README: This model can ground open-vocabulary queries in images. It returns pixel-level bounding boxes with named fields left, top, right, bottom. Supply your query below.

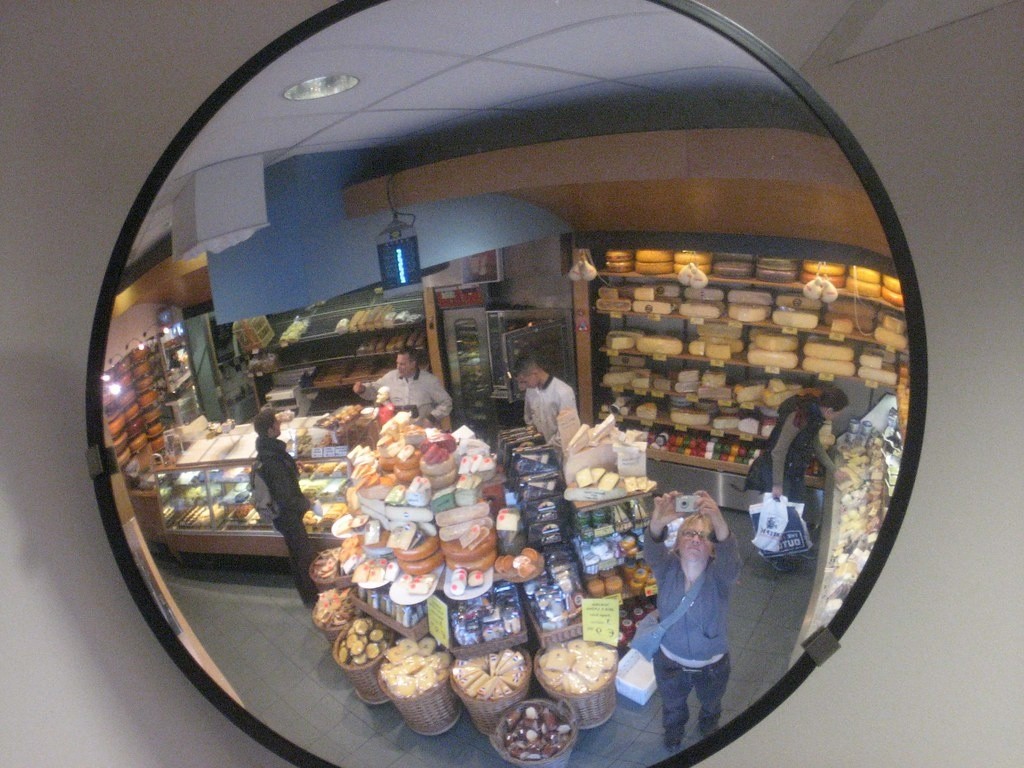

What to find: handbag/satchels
left=748, top=491, right=812, bottom=560
left=632, top=612, right=665, bottom=662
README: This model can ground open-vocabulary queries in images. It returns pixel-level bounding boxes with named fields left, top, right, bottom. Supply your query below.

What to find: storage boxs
left=617, top=448, right=647, bottom=477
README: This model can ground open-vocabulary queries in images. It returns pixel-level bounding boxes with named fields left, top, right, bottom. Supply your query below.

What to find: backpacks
left=249, top=452, right=285, bottom=523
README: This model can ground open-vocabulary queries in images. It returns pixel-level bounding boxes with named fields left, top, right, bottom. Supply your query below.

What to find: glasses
left=681, top=530, right=710, bottom=540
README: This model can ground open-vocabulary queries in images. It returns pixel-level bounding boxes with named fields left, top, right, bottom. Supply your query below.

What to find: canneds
left=844, top=418, right=873, bottom=446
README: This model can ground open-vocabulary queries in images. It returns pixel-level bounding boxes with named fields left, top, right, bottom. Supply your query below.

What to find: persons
left=232, top=319, right=273, bottom=403
left=169, top=351, right=181, bottom=370
left=254, top=410, right=319, bottom=606
left=353, top=347, right=453, bottom=426
left=744, top=387, right=849, bottom=573
left=644, top=489, right=738, bottom=751
left=514, top=356, right=581, bottom=448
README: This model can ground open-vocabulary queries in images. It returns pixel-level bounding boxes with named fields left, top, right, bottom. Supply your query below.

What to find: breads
left=278, top=304, right=426, bottom=356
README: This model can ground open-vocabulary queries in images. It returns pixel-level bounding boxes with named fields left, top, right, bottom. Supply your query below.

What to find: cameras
left=674, top=495, right=701, bottom=513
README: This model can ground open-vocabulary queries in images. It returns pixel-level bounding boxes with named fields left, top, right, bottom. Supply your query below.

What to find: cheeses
left=595, top=252, right=908, bottom=448
left=159, top=404, right=659, bottom=758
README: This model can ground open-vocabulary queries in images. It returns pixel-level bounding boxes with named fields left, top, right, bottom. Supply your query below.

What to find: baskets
left=308, top=552, right=619, bottom=768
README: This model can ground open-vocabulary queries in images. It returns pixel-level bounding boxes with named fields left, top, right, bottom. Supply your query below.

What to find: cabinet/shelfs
left=567, top=476, right=657, bottom=600
left=250, top=290, right=452, bottom=432
left=182, top=297, right=257, bottom=425
left=599, top=234, right=910, bottom=527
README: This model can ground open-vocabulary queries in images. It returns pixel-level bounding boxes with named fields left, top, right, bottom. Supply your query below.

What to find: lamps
left=377, top=175, right=425, bottom=300
